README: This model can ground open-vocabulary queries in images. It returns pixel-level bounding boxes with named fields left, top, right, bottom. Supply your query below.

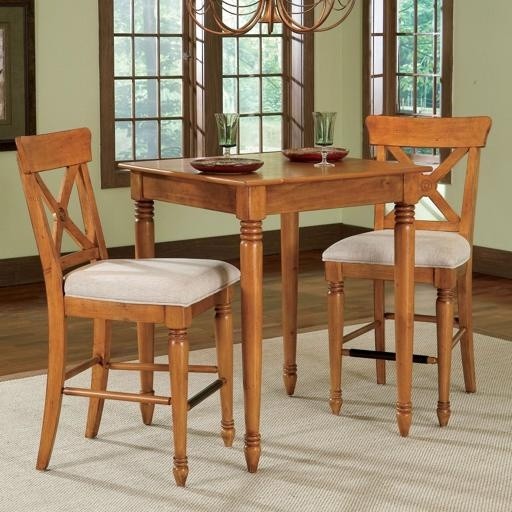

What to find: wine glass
left=312, top=111, right=336, bottom=169
left=215, top=111, right=240, bottom=156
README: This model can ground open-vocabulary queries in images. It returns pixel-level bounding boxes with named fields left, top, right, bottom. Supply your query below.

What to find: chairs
left=320, top=114, right=491, bottom=427
left=13, top=126, right=244, bottom=487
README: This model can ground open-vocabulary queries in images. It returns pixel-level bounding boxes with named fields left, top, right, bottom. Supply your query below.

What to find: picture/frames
left=0, top=1, right=35, bottom=151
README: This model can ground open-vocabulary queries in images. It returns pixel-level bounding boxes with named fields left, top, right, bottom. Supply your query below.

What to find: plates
left=190, top=157, right=264, bottom=175
left=281, top=146, right=350, bottom=162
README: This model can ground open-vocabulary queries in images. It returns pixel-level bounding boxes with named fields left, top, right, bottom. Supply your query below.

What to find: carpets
left=2, top=320, right=510, bottom=512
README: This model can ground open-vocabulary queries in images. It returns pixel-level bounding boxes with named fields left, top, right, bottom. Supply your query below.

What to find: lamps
left=180, top=0, right=358, bottom=39
